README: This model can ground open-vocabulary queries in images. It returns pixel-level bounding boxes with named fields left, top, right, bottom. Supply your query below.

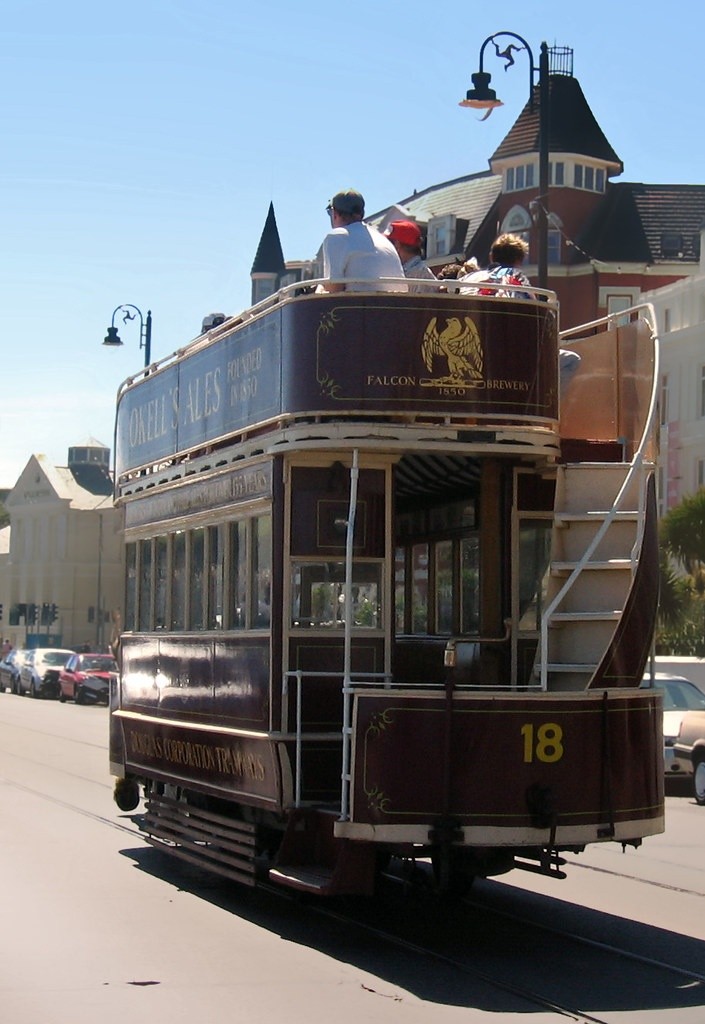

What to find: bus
left=110, top=277, right=663, bottom=899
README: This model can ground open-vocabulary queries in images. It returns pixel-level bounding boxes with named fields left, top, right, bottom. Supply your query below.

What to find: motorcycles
left=75, top=674, right=110, bottom=705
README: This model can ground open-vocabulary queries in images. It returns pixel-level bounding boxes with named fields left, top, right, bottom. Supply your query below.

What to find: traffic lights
left=0, top=603, right=59, bottom=626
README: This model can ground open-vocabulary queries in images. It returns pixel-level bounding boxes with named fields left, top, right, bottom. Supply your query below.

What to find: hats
left=382, top=220, right=420, bottom=248
left=325, top=189, right=365, bottom=217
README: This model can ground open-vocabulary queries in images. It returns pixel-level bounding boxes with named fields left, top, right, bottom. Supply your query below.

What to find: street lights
left=102, top=304, right=152, bottom=377
left=459, top=30, right=549, bottom=302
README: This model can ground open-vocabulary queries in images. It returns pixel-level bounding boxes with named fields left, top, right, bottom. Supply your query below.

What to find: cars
left=673, top=712, right=705, bottom=806
left=642, top=672, right=705, bottom=778
left=58, top=653, right=119, bottom=703
left=0, top=649, right=23, bottom=694
left=14, top=648, right=76, bottom=699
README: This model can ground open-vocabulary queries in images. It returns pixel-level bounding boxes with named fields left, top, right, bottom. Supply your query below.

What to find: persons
left=453, top=232, right=583, bottom=412
left=315, top=190, right=408, bottom=294
left=382, top=219, right=441, bottom=293
